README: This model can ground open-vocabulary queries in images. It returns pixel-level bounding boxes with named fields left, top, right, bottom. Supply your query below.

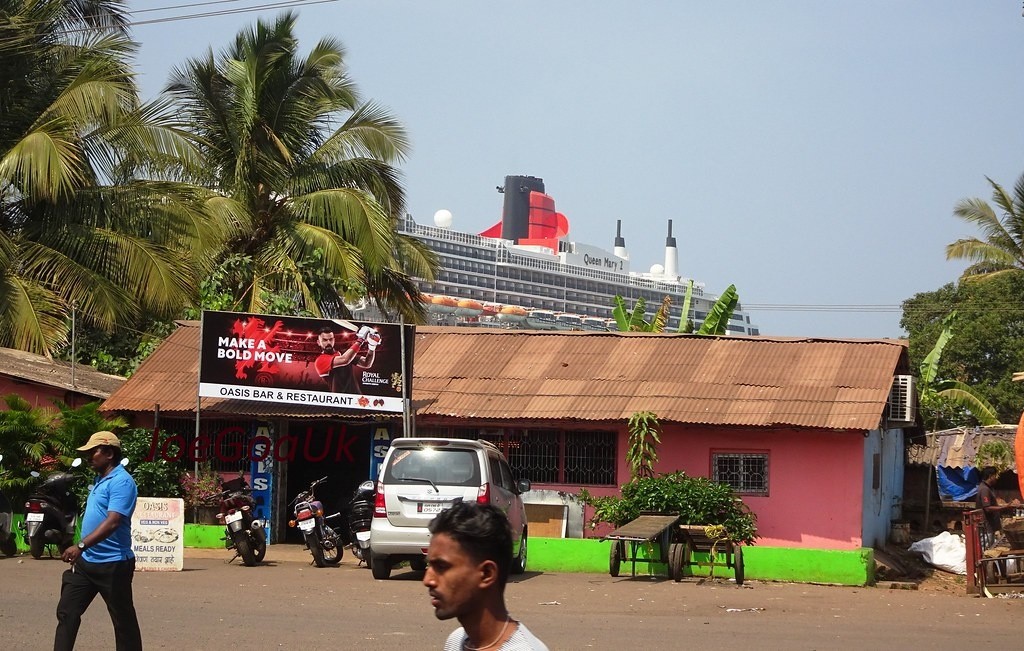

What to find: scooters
left=0, top=454, right=17, bottom=557
left=343, top=478, right=379, bottom=569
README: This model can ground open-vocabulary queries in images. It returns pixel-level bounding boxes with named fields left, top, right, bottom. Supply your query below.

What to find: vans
left=369, top=437, right=533, bottom=580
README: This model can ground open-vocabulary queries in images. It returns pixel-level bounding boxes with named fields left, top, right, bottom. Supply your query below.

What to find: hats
left=75, top=431, right=119, bottom=451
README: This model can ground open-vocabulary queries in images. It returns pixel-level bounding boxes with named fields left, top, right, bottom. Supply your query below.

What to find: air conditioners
left=884, top=375, right=916, bottom=423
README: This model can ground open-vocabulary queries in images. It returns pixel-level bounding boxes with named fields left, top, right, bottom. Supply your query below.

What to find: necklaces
left=463, top=613, right=511, bottom=651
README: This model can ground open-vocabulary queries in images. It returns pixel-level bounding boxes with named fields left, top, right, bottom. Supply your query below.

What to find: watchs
left=78, top=540, right=88, bottom=552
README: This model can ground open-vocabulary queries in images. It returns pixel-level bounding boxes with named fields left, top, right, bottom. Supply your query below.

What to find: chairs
left=402, top=465, right=472, bottom=484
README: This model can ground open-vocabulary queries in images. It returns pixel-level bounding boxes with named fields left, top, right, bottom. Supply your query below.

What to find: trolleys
left=599, top=510, right=681, bottom=579
left=673, top=523, right=746, bottom=586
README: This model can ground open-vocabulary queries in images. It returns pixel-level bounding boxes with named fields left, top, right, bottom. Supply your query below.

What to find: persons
left=976, top=466, right=1021, bottom=557
left=424, top=498, right=550, bottom=651
left=315, top=325, right=384, bottom=396
left=52, top=430, right=143, bottom=650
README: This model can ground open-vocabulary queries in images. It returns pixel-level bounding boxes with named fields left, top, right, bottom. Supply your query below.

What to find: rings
left=69, top=558, right=72, bottom=560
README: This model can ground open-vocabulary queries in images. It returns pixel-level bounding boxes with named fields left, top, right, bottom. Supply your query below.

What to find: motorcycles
left=17, top=457, right=82, bottom=560
left=288, top=475, right=344, bottom=569
left=205, top=454, right=268, bottom=566
